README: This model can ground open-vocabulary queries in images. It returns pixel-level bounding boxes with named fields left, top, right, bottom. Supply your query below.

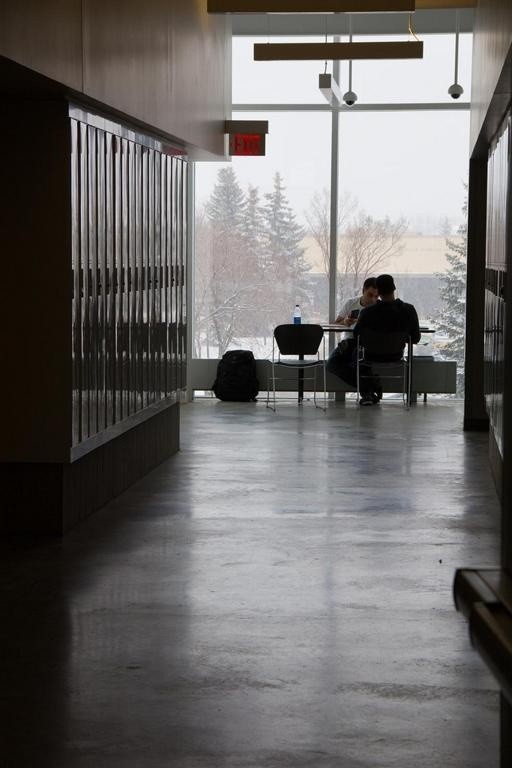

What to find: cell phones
left=351, top=310, right=359, bottom=324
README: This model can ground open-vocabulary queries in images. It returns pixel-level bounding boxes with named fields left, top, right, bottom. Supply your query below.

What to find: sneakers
left=359, top=391, right=380, bottom=406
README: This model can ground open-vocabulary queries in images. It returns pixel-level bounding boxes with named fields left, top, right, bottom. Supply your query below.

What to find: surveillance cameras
left=343, top=92, right=357, bottom=106
left=448, top=84, right=463, bottom=99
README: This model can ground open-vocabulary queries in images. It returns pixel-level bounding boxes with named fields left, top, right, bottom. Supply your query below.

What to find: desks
left=293, top=320, right=436, bottom=407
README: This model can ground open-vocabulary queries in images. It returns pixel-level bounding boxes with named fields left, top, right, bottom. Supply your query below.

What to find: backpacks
left=212, top=348, right=261, bottom=404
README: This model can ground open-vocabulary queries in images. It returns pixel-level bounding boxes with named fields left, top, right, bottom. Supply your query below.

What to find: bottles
left=293, top=305, right=302, bottom=325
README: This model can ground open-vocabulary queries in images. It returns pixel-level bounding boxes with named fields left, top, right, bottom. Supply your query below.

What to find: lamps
left=317, top=13, right=344, bottom=112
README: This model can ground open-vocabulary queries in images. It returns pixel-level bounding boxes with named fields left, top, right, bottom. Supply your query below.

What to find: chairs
left=265, top=323, right=328, bottom=414
left=353, top=320, right=413, bottom=409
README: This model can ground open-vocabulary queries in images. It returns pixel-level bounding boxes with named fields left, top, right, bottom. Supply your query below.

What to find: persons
left=328, top=275, right=421, bottom=405
left=334, top=278, right=378, bottom=344
